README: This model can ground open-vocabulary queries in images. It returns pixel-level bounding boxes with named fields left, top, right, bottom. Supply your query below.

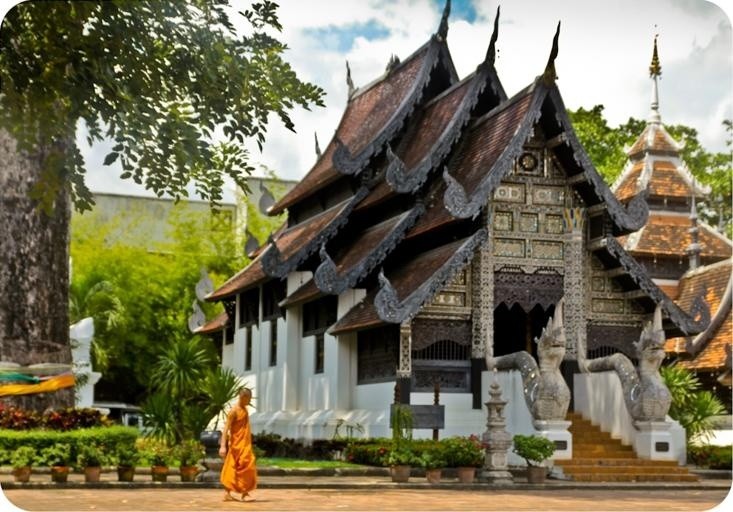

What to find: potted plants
left=10, top=439, right=206, bottom=482
left=388, top=433, right=558, bottom=484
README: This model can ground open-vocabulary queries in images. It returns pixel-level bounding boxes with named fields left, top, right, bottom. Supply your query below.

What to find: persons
left=219, top=388, right=257, bottom=502
